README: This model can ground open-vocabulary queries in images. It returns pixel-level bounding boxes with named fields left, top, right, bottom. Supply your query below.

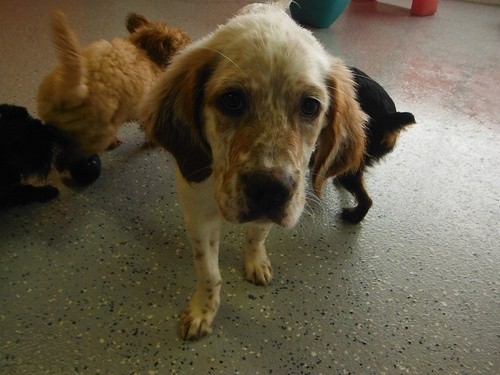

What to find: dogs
left=136, top=2, right=370, bottom=341
left=309, top=61, right=418, bottom=226
left=0, top=104, right=75, bottom=204
left=36, top=10, right=194, bottom=189
